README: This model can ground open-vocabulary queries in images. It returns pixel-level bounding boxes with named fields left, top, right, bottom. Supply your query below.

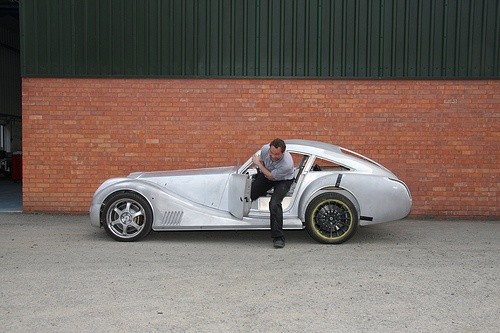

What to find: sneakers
left=274, top=238, right=285, bottom=248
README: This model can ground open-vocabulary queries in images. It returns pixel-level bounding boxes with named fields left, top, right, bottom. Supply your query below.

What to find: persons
left=251, top=138, right=296, bottom=249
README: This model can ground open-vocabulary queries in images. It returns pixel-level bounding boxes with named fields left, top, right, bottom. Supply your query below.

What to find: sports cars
left=89, top=138, right=413, bottom=245
